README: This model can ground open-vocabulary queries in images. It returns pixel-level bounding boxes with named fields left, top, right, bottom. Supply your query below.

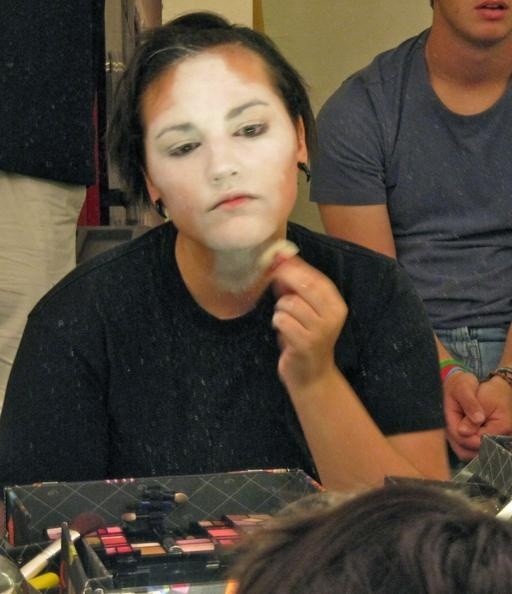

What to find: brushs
left=19, top=511, right=111, bottom=580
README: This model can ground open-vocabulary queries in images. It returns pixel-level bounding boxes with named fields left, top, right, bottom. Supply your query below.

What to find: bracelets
left=439, top=359, right=475, bottom=388
left=478, top=366, right=512, bottom=386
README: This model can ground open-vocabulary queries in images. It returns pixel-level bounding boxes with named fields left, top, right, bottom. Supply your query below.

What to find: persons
left=0, top=0, right=97, bottom=416
left=226, top=485, right=512, bottom=594
left=0, top=10, right=451, bottom=494
left=309, top=0, right=512, bottom=459
